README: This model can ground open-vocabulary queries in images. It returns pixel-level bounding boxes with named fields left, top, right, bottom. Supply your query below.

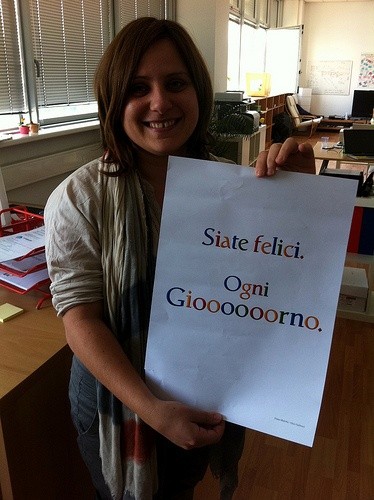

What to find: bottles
left=338, top=128, right=344, bottom=149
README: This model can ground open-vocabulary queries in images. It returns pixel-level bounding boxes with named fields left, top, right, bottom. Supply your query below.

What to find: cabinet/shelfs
left=0, top=205, right=53, bottom=310
left=248, top=92, right=294, bottom=150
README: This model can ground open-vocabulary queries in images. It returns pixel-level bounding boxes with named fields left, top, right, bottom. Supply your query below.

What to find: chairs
left=285, top=94, right=323, bottom=138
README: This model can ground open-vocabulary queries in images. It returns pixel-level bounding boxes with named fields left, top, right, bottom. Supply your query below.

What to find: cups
left=321, top=137, right=329, bottom=151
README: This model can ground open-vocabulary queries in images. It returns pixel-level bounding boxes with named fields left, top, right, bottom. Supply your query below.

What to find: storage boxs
left=339, top=267, right=369, bottom=312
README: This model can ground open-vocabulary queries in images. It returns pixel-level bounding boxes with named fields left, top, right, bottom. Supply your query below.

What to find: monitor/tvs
left=351, top=90, right=374, bottom=117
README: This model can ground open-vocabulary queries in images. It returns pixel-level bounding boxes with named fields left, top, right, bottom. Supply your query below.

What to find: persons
left=43, top=17, right=316, bottom=500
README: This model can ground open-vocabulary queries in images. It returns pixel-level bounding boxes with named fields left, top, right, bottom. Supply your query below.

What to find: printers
left=214, top=91, right=260, bottom=135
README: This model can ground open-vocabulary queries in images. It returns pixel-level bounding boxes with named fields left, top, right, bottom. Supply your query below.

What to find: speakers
left=343, top=125, right=374, bottom=154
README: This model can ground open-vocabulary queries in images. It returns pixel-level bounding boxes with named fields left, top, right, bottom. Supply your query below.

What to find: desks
left=317, top=117, right=369, bottom=129
left=213, top=125, right=266, bottom=168
left=313, top=142, right=374, bottom=175
left=0, top=280, right=101, bottom=500
